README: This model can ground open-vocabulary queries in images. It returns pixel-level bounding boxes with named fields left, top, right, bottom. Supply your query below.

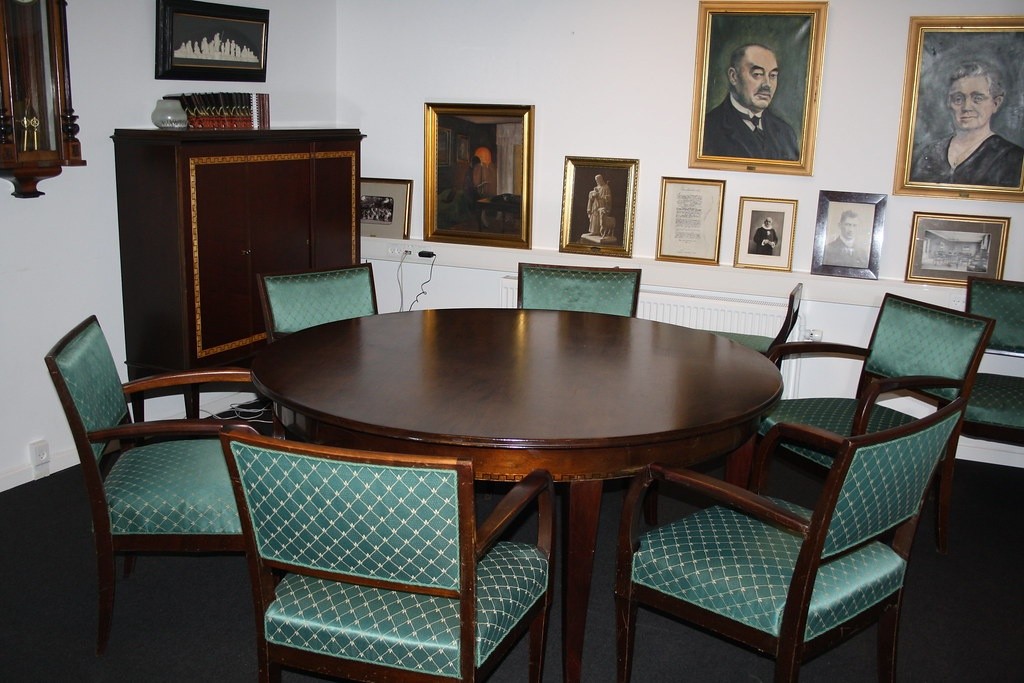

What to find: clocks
left=0, top=0, right=91, bottom=198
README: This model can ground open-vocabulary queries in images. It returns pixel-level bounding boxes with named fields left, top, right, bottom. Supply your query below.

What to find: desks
left=249, top=308, right=783, bottom=683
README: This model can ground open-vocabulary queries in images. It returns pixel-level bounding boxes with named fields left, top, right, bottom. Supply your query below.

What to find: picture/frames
left=689, top=0, right=829, bottom=176
left=810, top=190, right=887, bottom=279
left=906, top=211, right=1010, bottom=289
left=733, top=196, right=798, bottom=272
left=891, top=16, right=1024, bottom=204
left=422, top=103, right=536, bottom=251
left=360, top=177, right=414, bottom=239
left=559, top=156, right=640, bottom=258
left=155, top=0, right=269, bottom=82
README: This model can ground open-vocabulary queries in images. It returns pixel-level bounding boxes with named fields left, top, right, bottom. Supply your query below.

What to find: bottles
left=151, top=99, right=187, bottom=129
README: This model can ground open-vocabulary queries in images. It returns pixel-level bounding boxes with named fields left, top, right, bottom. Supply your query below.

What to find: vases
left=152, top=100, right=188, bottom=128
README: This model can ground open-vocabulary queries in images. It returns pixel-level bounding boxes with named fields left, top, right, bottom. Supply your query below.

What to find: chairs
left=46, top=262, right=1024, bottom=683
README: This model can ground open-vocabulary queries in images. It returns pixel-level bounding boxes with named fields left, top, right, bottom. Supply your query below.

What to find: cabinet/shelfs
left=110, top=128, right=366, bottom=398
